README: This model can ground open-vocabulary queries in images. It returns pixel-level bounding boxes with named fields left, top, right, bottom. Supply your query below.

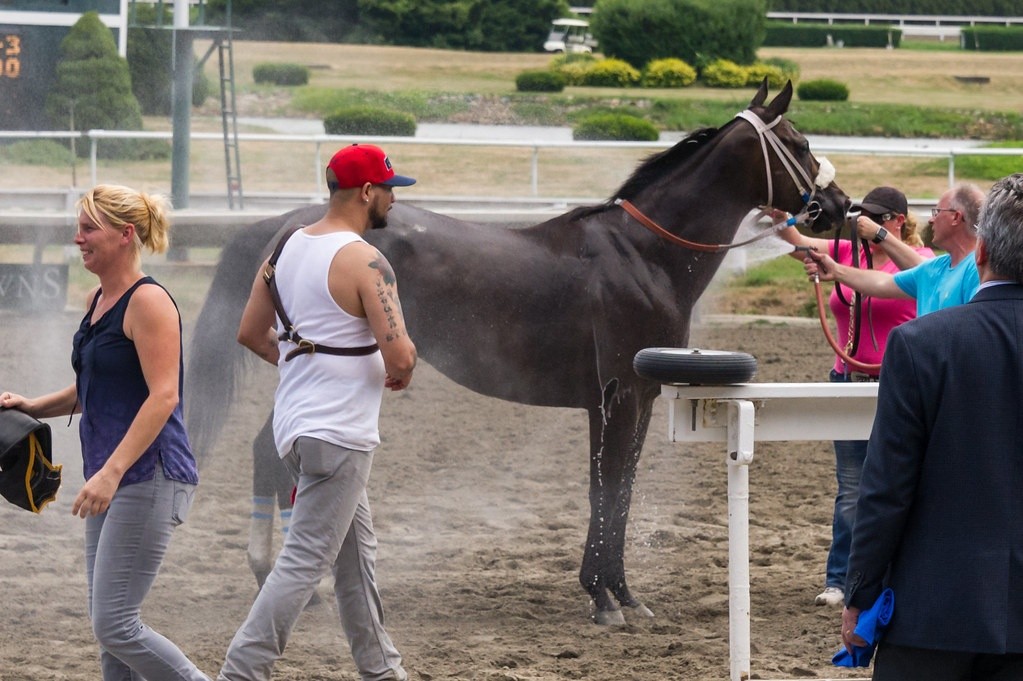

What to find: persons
left=0, top=183, right=213, bottom=681
left=842, top=172, right=1023, bottom=681
left=215, top=144, right=417, bottom=681
left=765, top=184, right=981, bottom=602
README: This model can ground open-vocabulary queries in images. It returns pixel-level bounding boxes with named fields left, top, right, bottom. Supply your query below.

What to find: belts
left=830, top=371, right=880, bottom=382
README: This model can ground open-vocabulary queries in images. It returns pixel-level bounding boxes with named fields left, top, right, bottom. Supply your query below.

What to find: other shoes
left=814, top=586, right=844, bottom=607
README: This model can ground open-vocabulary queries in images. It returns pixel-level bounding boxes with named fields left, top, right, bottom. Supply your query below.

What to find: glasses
left=932, top=209, right=964, bottom=221
left=868, top=215, right=895, bottom=226
left=971, top=223, right=980, bottom=232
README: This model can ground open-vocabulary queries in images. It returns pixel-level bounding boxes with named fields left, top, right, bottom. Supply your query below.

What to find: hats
left=847, top=188, right=908, bottom=217
left=325, top=143, right=417, bottom=189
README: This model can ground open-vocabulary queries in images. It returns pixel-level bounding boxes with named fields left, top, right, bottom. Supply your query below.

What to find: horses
left=184, top=75, right=853, bottom=628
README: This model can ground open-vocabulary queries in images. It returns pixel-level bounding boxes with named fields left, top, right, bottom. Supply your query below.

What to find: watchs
left=872, top=225, right=888, bottom=244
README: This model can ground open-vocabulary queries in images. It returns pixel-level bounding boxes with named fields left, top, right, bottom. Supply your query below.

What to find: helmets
left=0, top=406, right=62, bottom=513
